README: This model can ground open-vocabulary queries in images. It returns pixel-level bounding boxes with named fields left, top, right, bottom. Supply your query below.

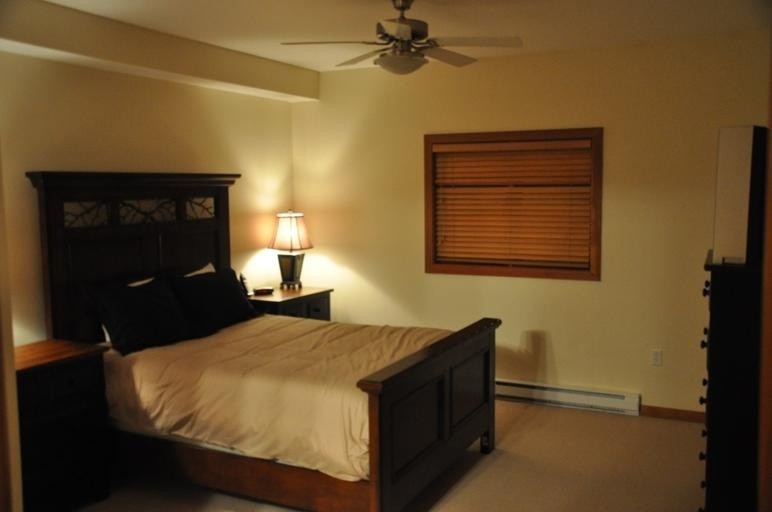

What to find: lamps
left=372, top=41, right=429, bottom=76
left=265, top=208, right=315, bottom=290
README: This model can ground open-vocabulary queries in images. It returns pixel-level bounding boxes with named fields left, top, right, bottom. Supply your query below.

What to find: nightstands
left=247, top=286, right=334, bottom=320
left=13, top=338, right=110, bottom=511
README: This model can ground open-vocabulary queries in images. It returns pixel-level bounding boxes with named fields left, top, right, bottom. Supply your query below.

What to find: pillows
left=88, top=263, right=265, bottom=356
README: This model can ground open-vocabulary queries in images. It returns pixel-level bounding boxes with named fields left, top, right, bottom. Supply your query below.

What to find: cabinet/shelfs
left=699, top=270, right=757, bottom=511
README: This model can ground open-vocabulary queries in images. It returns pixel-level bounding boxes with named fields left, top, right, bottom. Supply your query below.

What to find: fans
left=279, top=1, right=525, bottom=69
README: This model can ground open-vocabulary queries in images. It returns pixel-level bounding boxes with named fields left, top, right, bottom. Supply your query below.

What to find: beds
left=25, top=170, right=502, bottom=512
